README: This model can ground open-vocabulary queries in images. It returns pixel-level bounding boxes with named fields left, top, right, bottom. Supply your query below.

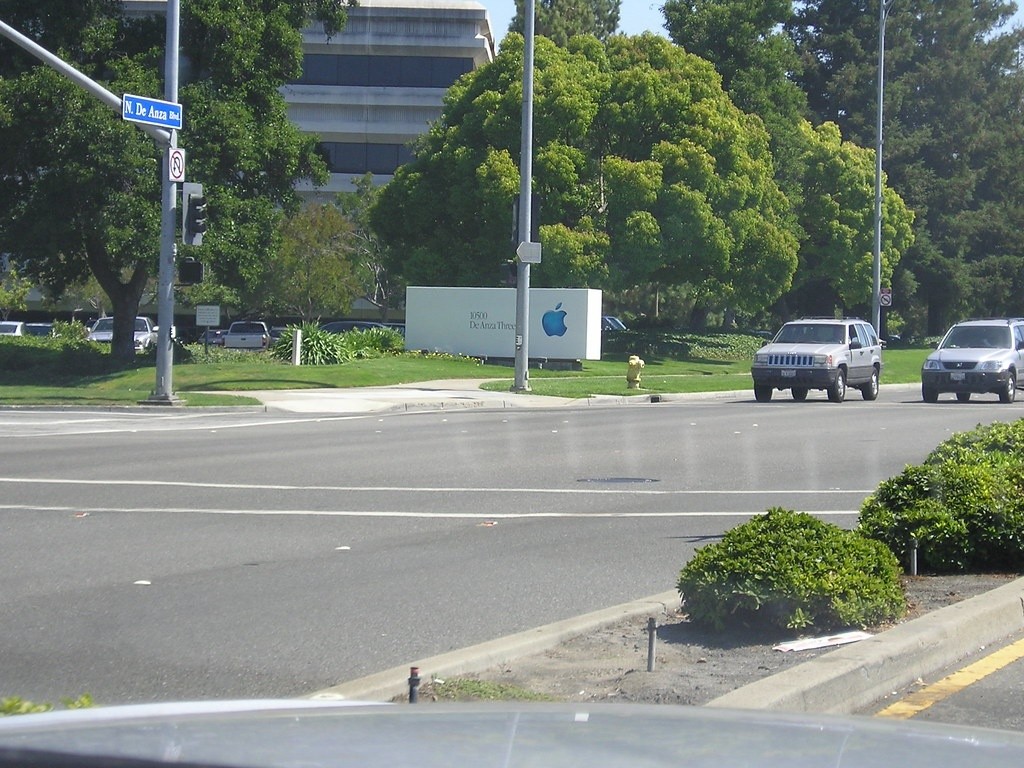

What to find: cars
left=879, top=339, right=887, bottom=350
left=608, top=317, right=627, bottom=330
left=320, top=321, right=385, bottom=334
left=268, top=327, right=287, bottom=346
left=601, top=317, right=617, bottom=331
left=747, top=329, right=772, bottom=338
left=0, top=321, right=23, bottom=336
left=88, top=317, right=159, bottom=352
left=198, top=330, right=215, bottom=344
left=214, top=330, right=228, bottom=345
left=889, top=335, right=900, bottom=340
left=27, top=324, right=53, bottom=336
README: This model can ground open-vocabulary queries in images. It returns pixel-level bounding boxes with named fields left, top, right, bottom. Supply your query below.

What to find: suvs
left=751, top=316, right=881, bottom=403
left=921, top=317, right=1024, bottom=404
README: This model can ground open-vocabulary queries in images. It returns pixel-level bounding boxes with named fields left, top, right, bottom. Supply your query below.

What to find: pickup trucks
left=225, top=322, right=270, bottom=352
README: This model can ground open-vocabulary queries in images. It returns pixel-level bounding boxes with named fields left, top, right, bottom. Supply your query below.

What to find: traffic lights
left=179, top=262, right=203, bottom=283
left=183, top=183, right=208, bottom=246
